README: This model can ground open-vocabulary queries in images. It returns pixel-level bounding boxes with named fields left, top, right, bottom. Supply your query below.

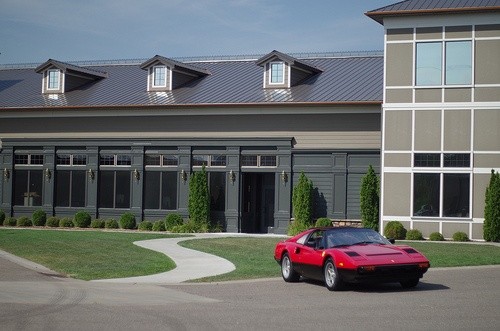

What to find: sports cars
left=274, top=226, right=431, bottom=291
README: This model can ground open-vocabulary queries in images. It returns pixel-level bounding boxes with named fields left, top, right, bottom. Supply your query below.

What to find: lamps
left=229, top=170, right=235, bottom=182
left=4, top=168, right=8, bottom=179
left=134, top=169, right=140, bottom=179
left=45, top=169, right=51, bottom=178
left=181, top=170, right=188, bottom=182
left=89, top=169, right=94, bottom=181
left=281, top=171, right=288, bottom=186
